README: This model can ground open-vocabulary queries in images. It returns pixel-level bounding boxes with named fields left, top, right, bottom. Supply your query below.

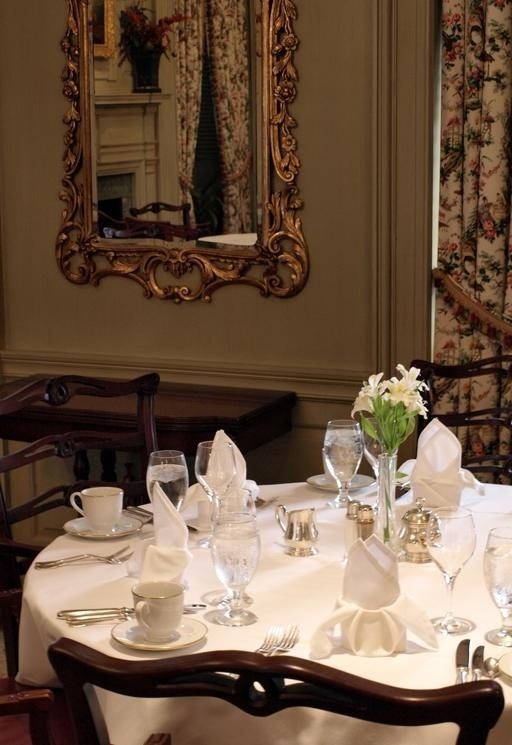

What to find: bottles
left=398, top=496, right=441, bottom=564
left=357, top=503, right=375, bottom=541
left=343, top=500, right=361, bottom=557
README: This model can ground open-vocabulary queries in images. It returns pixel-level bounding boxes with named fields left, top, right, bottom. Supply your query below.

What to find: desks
left=0, top=374, right=297, bottom=482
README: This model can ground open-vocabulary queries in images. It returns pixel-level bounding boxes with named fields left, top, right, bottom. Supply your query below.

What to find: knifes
left=125, top=505, right=198, bottom=533
left=471, top=646, right=485, bottom=681
left=456, top=638, right=470, bottom=685
left=57, top=603, right=207, bottom=619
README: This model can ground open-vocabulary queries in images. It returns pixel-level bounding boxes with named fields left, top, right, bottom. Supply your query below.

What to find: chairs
left=1, top=373, right=162, bottom=692
left=411, top=355, right=511, bottom=485
left=47, top=637, right=505, bottom=745
left=104, top=202, right=212, bottom=242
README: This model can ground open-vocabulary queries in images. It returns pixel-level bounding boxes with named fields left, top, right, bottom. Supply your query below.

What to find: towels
left=308, top=532, right=441, bottom=659
left=138, top=481, right=193, bottom=584
left=178, top=429, right=259, bottom=533
left=396, top=417, right=488, bottom=517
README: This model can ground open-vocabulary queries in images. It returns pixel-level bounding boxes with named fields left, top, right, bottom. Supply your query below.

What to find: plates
left=63, top=516, right=143, bottom=541
left=305, top=473, right=374, bottom=492
left=497, top=650, right=512, bottom=680
left=110, top=616, right=208, bottom=652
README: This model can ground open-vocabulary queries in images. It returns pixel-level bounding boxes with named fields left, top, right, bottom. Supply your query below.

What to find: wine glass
left=425, top=506, right=478, bottom=635
left=194, top=442, right=236, bottom=548
left=482, top=528, right=512, bottom=648
left=322, top=418, right=361, bottom=509
left=362, top=417, right=398, bottom=500
left=146, top=449, right=189, bottom=513
left=212, top=511, right=261, bottom=627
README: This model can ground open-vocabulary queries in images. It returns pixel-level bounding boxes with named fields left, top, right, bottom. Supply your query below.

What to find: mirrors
left=54, top=1, right=310, bottom=304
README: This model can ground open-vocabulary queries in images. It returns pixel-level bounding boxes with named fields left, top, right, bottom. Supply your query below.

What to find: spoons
left=484, top=656, right=500, bottom=681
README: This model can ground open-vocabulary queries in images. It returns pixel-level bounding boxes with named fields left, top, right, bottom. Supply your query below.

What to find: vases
left=129, top=44, right=166, bottom=92
left=378, top=454, right=406, bottom=561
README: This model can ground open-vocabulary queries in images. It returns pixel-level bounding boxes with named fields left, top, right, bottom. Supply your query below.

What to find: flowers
left=350, top=363, right=431, bottom=543
left=115, top=0, right=190, bottom=68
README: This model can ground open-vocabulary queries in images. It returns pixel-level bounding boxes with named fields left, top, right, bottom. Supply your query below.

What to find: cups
left=322, top=450, right=338, bottom=483
left=275, top=504, right=319, bottom=556
left=69, top=486, right=125, bottom=535
left=131, top=581, right=186, bottom=642
left=197, top=498, right=214, bottom=523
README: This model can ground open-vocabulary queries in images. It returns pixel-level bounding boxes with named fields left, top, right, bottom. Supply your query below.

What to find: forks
left=35, top=545, right=133, bottom=570
left=256, top=624, right=303, bottom=657
